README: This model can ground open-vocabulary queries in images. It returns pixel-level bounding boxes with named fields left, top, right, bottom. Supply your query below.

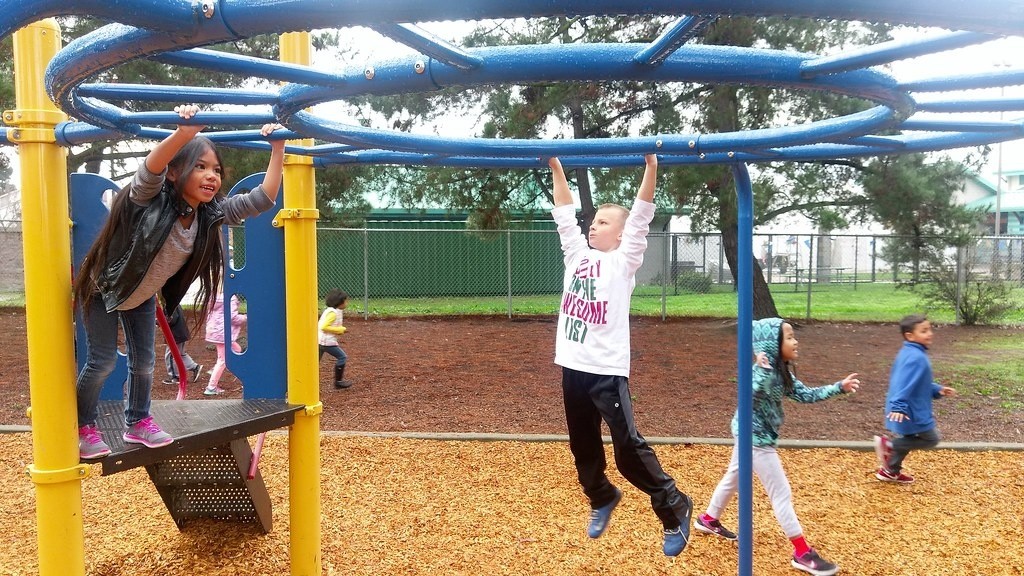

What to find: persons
left=155, top=303, right=204, bottom=384
left=76, top=104, right=285, bottom=459
left=694, top=317, right=860, bottom=576
left=203, top=269, right=248, bottom=395
left=318, top=289, right=352, bottom=389
left=536, top=154, right=693, bottom=557
left=874, top=315, right=957, bottom=483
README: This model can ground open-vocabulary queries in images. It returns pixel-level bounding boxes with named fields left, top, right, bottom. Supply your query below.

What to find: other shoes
left=193, top=364, right=204, bottom=383
left=203, top=386, right=226, bottom=395
left=205, top=369, right=214, bottom=375
left=162, top=376, right=179, bottom=385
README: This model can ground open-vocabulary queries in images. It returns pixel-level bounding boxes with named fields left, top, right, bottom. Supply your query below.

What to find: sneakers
left=791, top=547, right=839, bottom=576
left=873, top=434, right=894, bottom=470
left=694, top=514, right=738, bottom=541
left=123, top=415, right=175, bottom=448
left=78, top=423, right=113, bottom=459
left=588, top=487, right=623, bottom=538
left=662, top=495, right=693, bottom=557
left=876, top=464, right=914, bottom=483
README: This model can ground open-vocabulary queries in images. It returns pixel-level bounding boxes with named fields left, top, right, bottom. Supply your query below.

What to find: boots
left=335, top=364, right=352, bottom=389
